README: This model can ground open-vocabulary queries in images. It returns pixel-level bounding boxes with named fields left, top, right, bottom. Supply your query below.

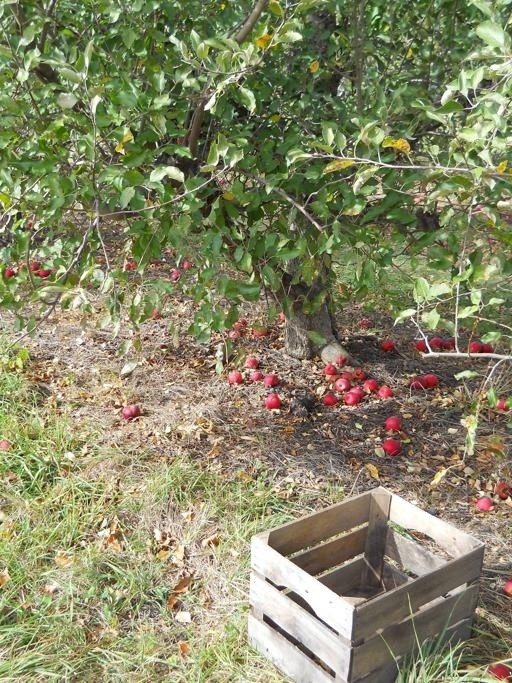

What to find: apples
left=172, top=261, right=189, bottom=283
left=382, top=439, right=400, bottom=455
left=487, top=662, right=512, bottom=683
left=361, top=320, right=370, bottom=329
left=503, top=578, right=512, bottom=597
left=122, top=404, right=139, bottom=420
left=386, top=416, right=401, bottom=431
left=416, top=337, right=454, bottom=351
left=6, top=271, right=13, bottom=277
left=495, top=398, right=504, bottom=409
left=152, top=309, right=160, bottom=320
left=323, top=356, right=393, bottom=405
left=477, top=496, right=492, bottom=511
left=32, top=262, right=50, bottom=278
left=471, top=341, right=492, bottom=353
left=495, top=481, right=512, bottom=501
left=266, top=393, right=281, bottom=409
left=229, top=358, right=278, bottom=388
left=126, top=260, right=136, bottom=270
left=382, top=340, right=394, bottom=351
left=229, top=318, right=269, bottom=338
left=279, top=312, right=284, bottom=321
left=410, top=375, right=438, bottom=389
